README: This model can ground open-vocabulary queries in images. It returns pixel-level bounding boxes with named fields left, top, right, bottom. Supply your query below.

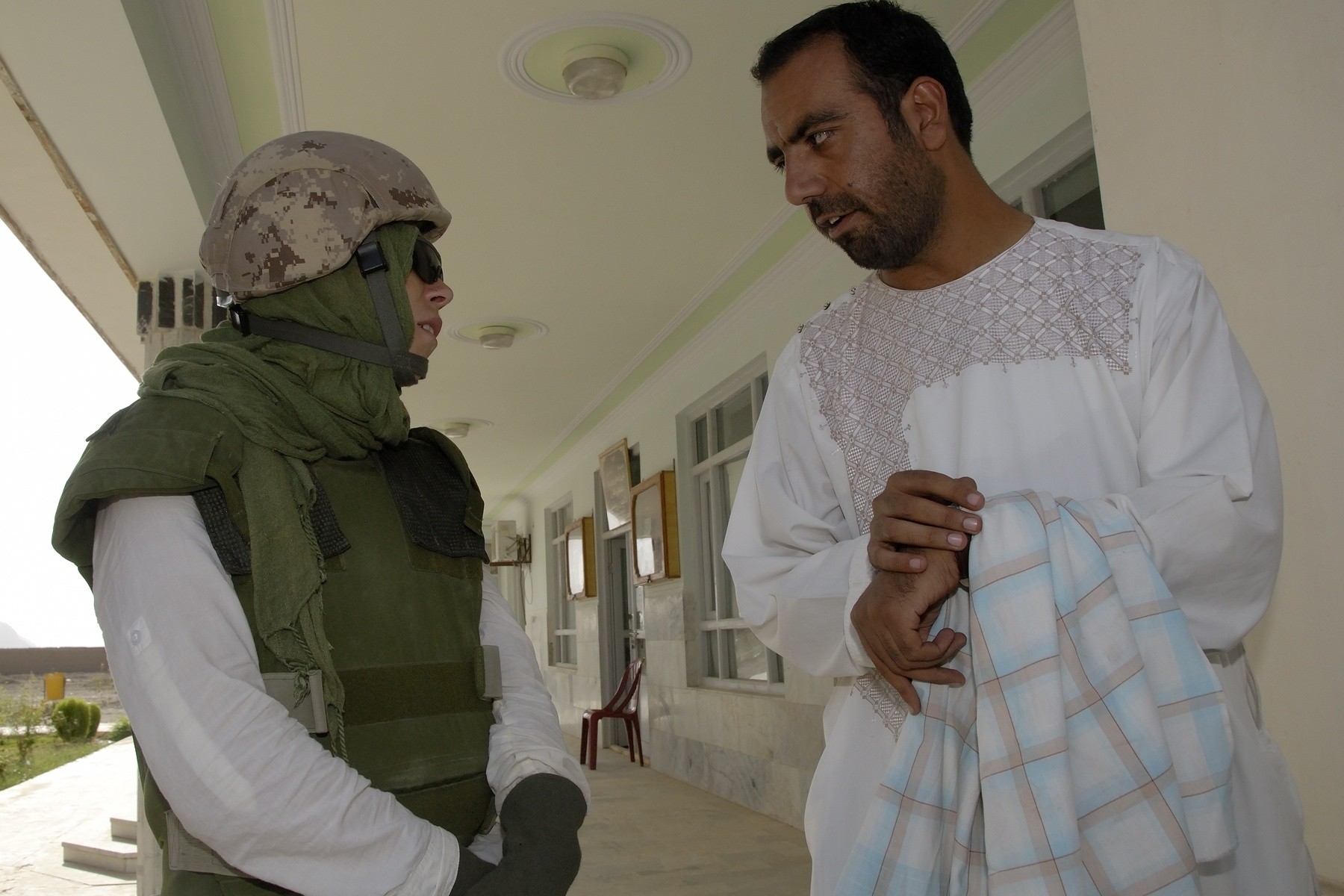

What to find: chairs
left=580, top=661, right=644, bottom=770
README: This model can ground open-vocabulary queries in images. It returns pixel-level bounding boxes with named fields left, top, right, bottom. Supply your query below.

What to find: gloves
left=496, top=773, right=588, bottom=896
left=447, top=844, right=497, bottom=896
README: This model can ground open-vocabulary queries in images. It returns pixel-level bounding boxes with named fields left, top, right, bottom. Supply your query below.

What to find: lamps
left=443, top=423, right=469, bottom=438
left=562, top=46, right=628, bottom=100
left=478, top=326, right=514, bottom=349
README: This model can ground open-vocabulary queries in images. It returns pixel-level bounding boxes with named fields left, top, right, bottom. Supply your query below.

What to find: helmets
left=197, top=131, right=454, bottom=300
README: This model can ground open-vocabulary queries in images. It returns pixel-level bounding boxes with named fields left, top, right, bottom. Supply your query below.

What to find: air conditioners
left=491, top=521, right=518, bottom=563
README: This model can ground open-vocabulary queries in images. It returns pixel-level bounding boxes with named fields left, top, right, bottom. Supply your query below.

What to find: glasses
left=412, top=236, right=447, bottom=284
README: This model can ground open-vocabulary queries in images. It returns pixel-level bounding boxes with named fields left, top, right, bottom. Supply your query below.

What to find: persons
left=718, top=0, right=1316, bottom=896
left=52, top=133, right=589, bottom=896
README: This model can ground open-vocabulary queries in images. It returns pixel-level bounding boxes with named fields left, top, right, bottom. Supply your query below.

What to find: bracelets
left=941, top=503, right=971, bottom=580
